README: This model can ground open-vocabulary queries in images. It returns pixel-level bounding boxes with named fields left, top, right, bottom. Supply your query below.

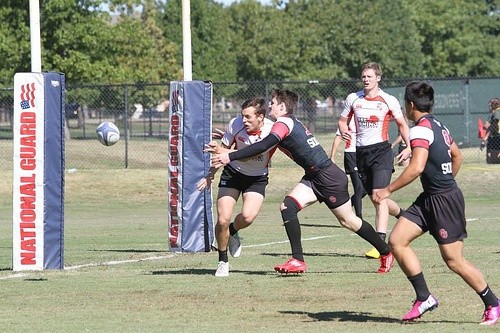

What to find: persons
left=202, top=89, right=394, bottom=273
left=331, top=115, right=368, bottom=218
left=339, top=63, right=410, bottom=259
left=481, top=97, right=500, bottom=163
left=372, top=82, right=500, bottom=325
left=398, top=130, right=407, bottom=166
left=198, top=97, right=277, bottom=277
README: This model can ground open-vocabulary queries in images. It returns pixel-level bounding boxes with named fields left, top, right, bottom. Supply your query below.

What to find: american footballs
left=95, top=122, right=121, bottom=147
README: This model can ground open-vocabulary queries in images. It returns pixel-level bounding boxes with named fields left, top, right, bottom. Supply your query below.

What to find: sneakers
left=214, top=261, right=229, bottom=277
left=229, top=232, right=242, bottom=258
left=365, top=247, right=380, bottom=258
left=479, top=300, right=500, bottom=325
left=274, top=257, right=306, bottom=275
left=378, top=251, right=394, bottom=272
left=402, top=293, right=438, bottom=321
